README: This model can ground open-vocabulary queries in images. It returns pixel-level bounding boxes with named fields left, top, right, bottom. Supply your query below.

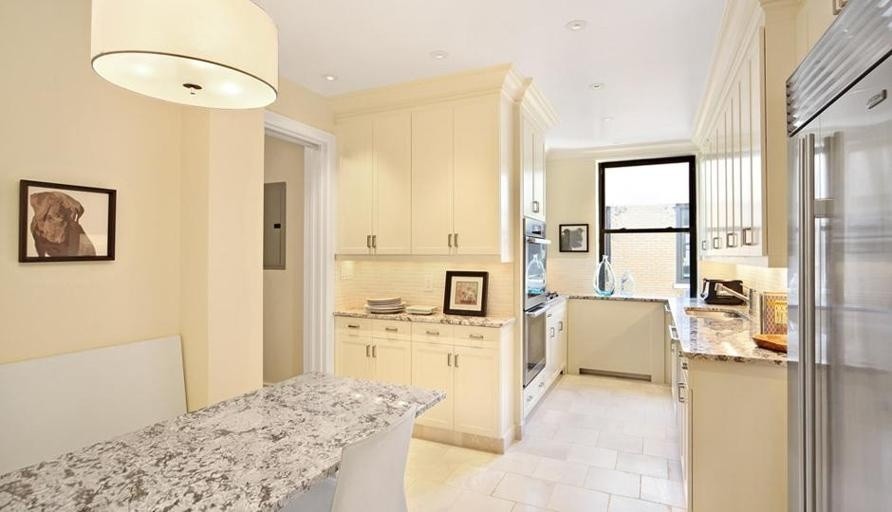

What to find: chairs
left=285, top=405, right=416, bottom=512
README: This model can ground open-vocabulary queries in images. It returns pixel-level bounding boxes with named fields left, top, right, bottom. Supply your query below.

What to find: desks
left=1, top=370, right=448, bottom=512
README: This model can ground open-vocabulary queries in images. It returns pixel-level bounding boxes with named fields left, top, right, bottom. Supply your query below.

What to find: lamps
left=89, top=0, right=280, bottom=111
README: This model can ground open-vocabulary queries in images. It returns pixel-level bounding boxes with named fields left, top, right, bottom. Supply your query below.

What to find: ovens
left=522, top=215, right=552, bottom=389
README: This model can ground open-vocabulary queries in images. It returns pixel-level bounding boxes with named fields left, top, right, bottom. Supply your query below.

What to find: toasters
left=700, top=277, right=745, bottom=305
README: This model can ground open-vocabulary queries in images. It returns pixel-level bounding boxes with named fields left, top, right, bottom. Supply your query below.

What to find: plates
left=364, top=297, right=405, bottom=314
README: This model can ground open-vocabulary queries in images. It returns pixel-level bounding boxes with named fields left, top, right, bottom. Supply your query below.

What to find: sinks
left=683, top=306, right=747, bottom=319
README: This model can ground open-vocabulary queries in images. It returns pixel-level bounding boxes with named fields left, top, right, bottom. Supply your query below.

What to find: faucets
left=714, top=283, right=758, bottom=315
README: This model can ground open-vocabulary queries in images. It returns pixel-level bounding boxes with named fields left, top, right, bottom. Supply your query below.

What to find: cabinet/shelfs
left=411, top=316, right=517, bottom=456
left=411, top=93, right=515, bottom=265
left=333, top=311, right=411, bottom=386
left=334, top=111, right=412, bottom=260
left=695, top=26, right=789, bottom=268
left=665, top=299, right=791, bottom=511
left=516, top=107, right=547, bottom=222
left=546, top=302, right=567, bottom=376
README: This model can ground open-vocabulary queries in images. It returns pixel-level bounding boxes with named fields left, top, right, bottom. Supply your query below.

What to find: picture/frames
left=558, top=223, right=589, bottom=253
left=18, top=178, right=117, bottom=265
left=443, top=270, right=489, bottom=317
